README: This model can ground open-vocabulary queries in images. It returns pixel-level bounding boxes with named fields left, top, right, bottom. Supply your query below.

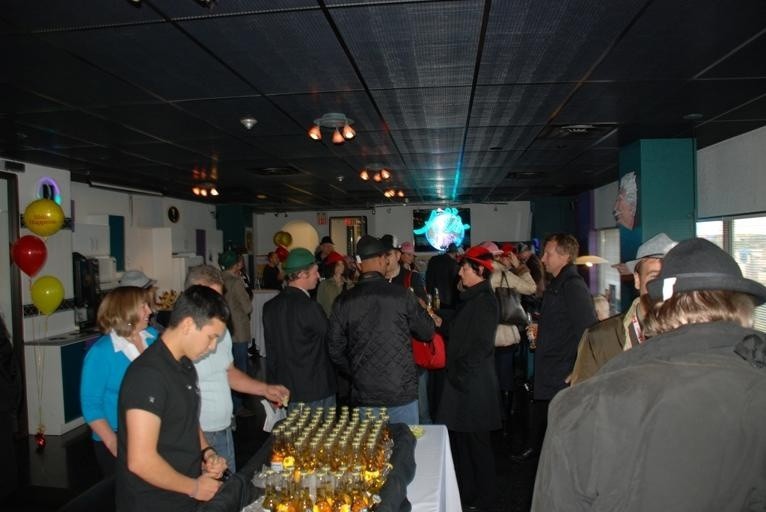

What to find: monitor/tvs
left=413, top=208, right=470, bottom=251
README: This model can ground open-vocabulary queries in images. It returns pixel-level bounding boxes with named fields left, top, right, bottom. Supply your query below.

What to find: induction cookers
left=48, top=330, right=86, bottom=341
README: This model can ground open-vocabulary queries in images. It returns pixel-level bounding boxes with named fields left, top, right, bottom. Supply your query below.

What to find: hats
left=627, top=231, right=679, bottom=272
left=282, top=233, right=531, bottom=272
left=120, top=269, right=158, bottom=290
left=645, top=237, right=766, bottom=304
left=218, top=250, right=236, bottom=266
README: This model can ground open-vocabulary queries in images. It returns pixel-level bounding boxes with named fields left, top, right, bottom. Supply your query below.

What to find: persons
left=571, top=234, right=678, bottom=385
left=185, top=266, right=291, bottom=472
left=259, top=235, right=436, bottom=425
left=81, top=272, right=166, bottom=512
left=113, top=284, right=231, bottom=512
left=530, top=238, right=766, bottom=512
left=613, top=174, right=637, bottom=228
left=426, top=232, right=610, bottom=494
left=218, top=248, right=254, bottom=374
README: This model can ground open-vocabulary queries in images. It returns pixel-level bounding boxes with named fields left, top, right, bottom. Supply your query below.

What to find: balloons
left=10, top=237, right=49, bottom=278
left=30, top=277, right=65, bottom=317
left=24, top=198, right=66, bottom=236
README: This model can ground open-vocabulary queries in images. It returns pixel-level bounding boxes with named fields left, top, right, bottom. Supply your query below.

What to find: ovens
left=61, top=338, right=101, bottom=424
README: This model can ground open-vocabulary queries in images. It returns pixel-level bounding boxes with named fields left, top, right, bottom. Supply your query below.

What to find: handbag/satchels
left=410, top=331, right=446, bottom=369
left=495, top=285, right=529, bottom=325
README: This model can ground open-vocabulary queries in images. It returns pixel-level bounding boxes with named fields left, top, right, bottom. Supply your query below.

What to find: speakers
left=215, top=205, right=253, bottom=228
left=534, top=197, right=576, bottom=234
left=223, top=228, right=245, bottom=254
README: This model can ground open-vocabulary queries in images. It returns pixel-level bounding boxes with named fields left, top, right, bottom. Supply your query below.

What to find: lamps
left=191, top=182, right=219, bottom=197
left=359, top=162, right=392, bottom=182
left=307, top=112, right=356, bottom=143
left=572, top=255, right=609, bottom=268
left=381, top=181, right=408, bottom=200
left=369, top=202, right=509, bottom=217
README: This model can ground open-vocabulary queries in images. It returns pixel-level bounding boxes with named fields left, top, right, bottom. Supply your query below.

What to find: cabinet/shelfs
left=70, top=212, right=219, bottom=307
left=615, top=135, right=699, bottom=308
left=24, top=329, right=102, bottom=437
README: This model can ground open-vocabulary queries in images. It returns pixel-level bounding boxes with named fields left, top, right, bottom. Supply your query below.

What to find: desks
left=191, top=407, right=467, bottom=511
left=246, top=287, right=288, bottom=360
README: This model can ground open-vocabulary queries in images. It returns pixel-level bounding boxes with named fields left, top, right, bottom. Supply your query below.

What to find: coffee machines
left=73, top=253, right=118, bottom=330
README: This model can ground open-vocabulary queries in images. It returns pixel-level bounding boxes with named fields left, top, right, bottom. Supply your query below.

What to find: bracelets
left=202, top=447, right=214, bottom=463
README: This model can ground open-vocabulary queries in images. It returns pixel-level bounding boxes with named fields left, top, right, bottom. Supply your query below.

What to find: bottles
left=433, top=288, right=441, bottom=309
left=255, top=280, right=261, bottom=292
left=527, top=311, right=539, bottom=349
left=605, top=284, right=615, bottom=303
left=425, top=295, right=433, bottom=312
left=261, top=401, right=392, bottom=512
